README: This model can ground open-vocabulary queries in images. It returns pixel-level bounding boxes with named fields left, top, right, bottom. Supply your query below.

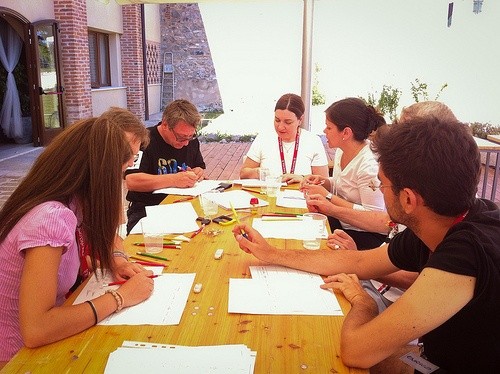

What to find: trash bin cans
left=10, top=117, right=33, bottom=143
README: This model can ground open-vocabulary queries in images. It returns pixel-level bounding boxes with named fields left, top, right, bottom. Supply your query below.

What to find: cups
left=201, top=190, right=220, bottom=216
left=302, top=213, right=327, bottom=250
left=141, top=217, right=164, bottom=254
left=258, top=167, right=281, bottom=197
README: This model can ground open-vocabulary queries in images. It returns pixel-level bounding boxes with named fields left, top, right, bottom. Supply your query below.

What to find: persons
left=126, top=99, right=209, bottom=235
left=240, top=93, right=329, bottom=185
left=0, top=117, right=155, bottom=370
left=78, top=107, right=150, bottom=240
left=232, top=115, right=500, bottom=374
left=299, top=98, right=391, bottom=250
left=327, top=102, right=457, bottom=313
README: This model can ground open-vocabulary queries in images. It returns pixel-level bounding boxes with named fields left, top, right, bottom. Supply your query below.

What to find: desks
left=481, top=134, right=500, bottom=199
left=472, top=136, right=500, bottom=201
left=0, top=180, right=370, bottom=374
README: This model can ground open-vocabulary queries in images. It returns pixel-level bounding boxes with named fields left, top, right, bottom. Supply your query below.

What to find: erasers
left=194, top=284, right=202, bottom=293
left=214, top=249, right=224, bottom=259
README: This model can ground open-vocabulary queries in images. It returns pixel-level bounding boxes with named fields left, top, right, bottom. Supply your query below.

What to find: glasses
left=167, top=122, right=198, bottom=142
left=133, top=153, right=140, bottom=162
left=379, top=182, right=419, bottom=202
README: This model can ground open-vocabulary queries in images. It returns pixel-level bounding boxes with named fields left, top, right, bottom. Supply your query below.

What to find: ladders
left=160, top=52, right=175, bottom=112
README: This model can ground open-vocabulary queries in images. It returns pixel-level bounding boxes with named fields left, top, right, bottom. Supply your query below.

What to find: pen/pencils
left=261, top=212, right=303, bottom=221
left=178, top=166, right=200, bottom=182
left=102, top=275, right=161, bottom=287
left=301, top=175, right=310, bottom=182
left=191, top=223, right=205, bottom=238
left=173, top=197, right=194, bottom=203
left=129, top=256, right=149, bottom=261
left=131, top=243, right=180, bottom=245
left=136, top=244, right=182, bottom=249
left=132, top=261, right=167, bottom=267
left=228, top=200, right=248, bottom=238
left=136, top=252, right=171, bottom=262
left=242, top=188, right=260, bottom=194
left=283, top=196, right=306, bottom=200
left=163, top=237, right=189, bottom=242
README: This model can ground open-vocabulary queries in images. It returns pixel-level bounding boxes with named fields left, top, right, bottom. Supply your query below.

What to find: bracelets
left=109, top=250, right=129, bottom=261
left=84, top=300, right=98, bottom=326
left=106, top=289, right=124, bottom=313
left=325, top=192, right=332, bottom=200
